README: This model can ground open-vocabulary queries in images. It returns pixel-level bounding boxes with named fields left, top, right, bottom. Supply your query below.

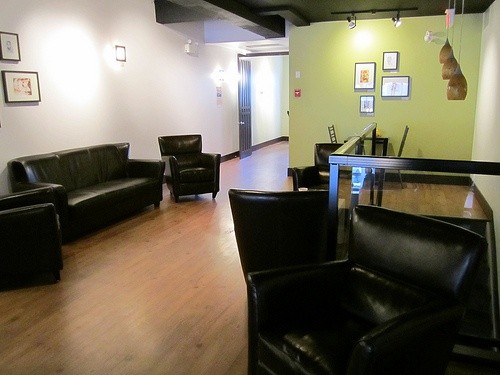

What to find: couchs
left=11, top=142, right=166, bottom=243
left=157, top=134, right=221, bottom=201
left=0, top=186, right=64, bottom=291
left=228, top=140, right=500, bottom=375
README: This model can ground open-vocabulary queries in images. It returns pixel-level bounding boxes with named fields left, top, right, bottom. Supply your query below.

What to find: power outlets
left=216, top=87, right=221, bottom=97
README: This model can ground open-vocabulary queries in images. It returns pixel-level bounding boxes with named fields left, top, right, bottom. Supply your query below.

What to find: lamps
left=423, top=0, right=467, bottom=100
left=391, top=11, right=401, bottom=28
left=184, top=40, right=199, bottom=57
left=347, top=14, right=357, bottom=29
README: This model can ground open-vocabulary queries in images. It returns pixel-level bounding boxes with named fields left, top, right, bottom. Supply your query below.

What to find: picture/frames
left=1, top=70, right=40, bottom=102
left=360, top=95, right=375, bottom=113
left=354, top=62, right=376, bottom=91
left=381, top=76, right=411, bottom=97
left=382, top=52, right=400, bottom=71
left=0, top=31, right=20, bottom=61
